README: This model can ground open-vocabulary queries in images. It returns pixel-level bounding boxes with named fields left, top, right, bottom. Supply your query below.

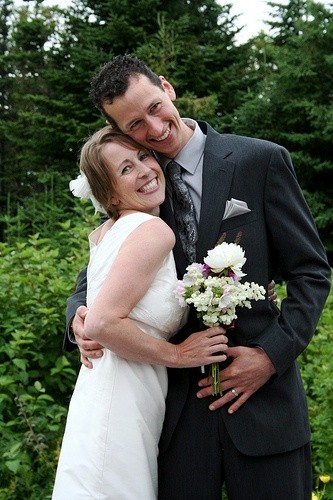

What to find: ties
left=165, top=160, right=198, bottom=264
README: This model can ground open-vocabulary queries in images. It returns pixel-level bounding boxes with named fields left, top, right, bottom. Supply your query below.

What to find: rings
left=231, top=388, right=239, bottom=397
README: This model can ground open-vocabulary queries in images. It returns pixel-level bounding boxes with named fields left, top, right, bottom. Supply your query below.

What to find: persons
left=57, top=50, right=333, bottom=500
left=49, top=124, right=229, bottom=500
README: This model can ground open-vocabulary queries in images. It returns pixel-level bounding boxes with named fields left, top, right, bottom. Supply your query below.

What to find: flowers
left=68, top=168, right=110, bottom=217
left=172, top=241, right=266, bottom=395
